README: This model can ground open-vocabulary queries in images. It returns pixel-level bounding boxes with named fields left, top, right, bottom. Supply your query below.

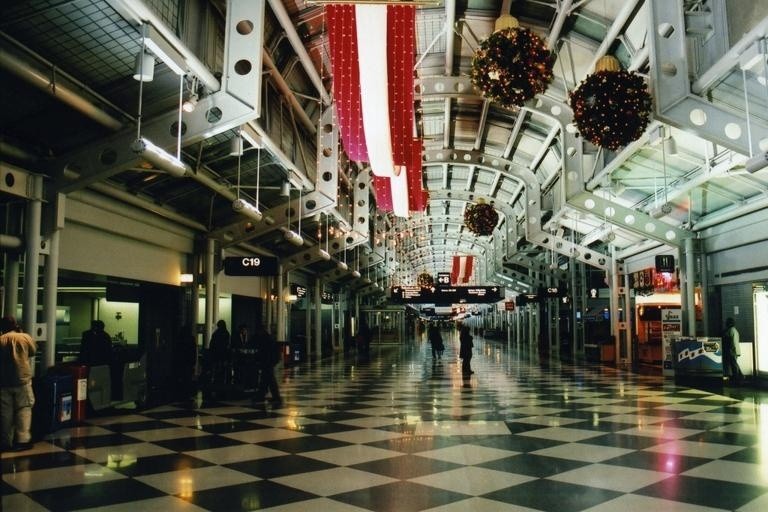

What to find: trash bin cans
left=281, top=342, right=302, bottom=362
left=37, top=364, right=88, bottom=433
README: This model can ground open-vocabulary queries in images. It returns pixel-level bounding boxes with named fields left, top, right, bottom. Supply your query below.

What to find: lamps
left=569, top=251, right=580, bottom=260
left=352, top=271, right=360, bottom=278
left=653, top=203, right=671, bottom=219
left=132, top=138, right=186, bottom=177
left=317, top=248, right=331, bottom=262
left=601, top=231, right=615, bottom=244
left=183, top=94, right=197, bottom=112
left=281, top=182, right=291, bottom=196
left=745, top=151, right=768, bottom=174
left=284, top=231, right=303, bottom=247
left=667, top=135, right=680, bottom=157
left=337, top=262, right=348, bottom=271
left=133, top=52, right=155, bottom=83
left=229, top=136, right=244, bottom=157
left=564, top=56, right=654, bottom=152
left=232, top=199, right=262, bottom=221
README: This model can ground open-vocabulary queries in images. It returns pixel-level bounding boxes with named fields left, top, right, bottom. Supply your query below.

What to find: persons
left=232, top=327, right=249, bottom=386
left=427, top=322, right=445, bottom=360
left=250, top=326, right=281, bottom=403
left=82, top=321, right=111, bottom=371
left=209, top=319, right=228, bottom=384
left=459, top=326, right=476, bottom=376
left=0, top=314, right=40, bottom=450
left=724, top=316, right=743, bottom=383
left=416, top=319, right=455, bottom=341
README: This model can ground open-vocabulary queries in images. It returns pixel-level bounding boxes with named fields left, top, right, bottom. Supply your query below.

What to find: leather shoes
left=14, top=443, right=32, bottom=451
